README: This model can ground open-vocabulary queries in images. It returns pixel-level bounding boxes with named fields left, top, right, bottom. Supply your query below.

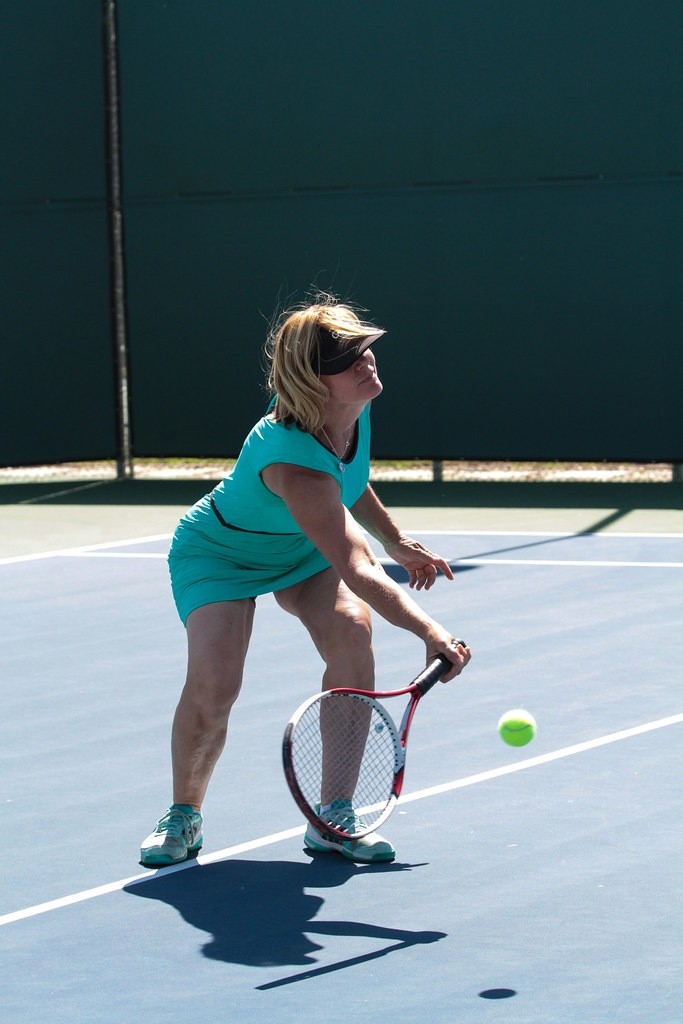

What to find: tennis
left=499, top=709, right=535, bottom=747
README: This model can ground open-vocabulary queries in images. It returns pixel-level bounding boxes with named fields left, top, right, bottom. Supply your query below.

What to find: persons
left=140, top=305, right=472, bottom=867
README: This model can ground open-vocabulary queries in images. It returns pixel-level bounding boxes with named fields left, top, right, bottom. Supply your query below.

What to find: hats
left=309, top=326, right=387, bottom=376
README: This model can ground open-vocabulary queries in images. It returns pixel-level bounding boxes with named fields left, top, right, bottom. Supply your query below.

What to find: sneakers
left=141, top=804, right=203, bottom=865
left=304, top=797, right=395, bottom=863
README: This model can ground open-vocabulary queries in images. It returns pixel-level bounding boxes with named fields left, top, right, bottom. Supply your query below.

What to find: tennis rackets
left=282, top=638, right=468, bottom=838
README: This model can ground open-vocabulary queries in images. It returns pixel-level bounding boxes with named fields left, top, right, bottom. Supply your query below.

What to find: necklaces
left=321, top=425, right=351, bottom=472
